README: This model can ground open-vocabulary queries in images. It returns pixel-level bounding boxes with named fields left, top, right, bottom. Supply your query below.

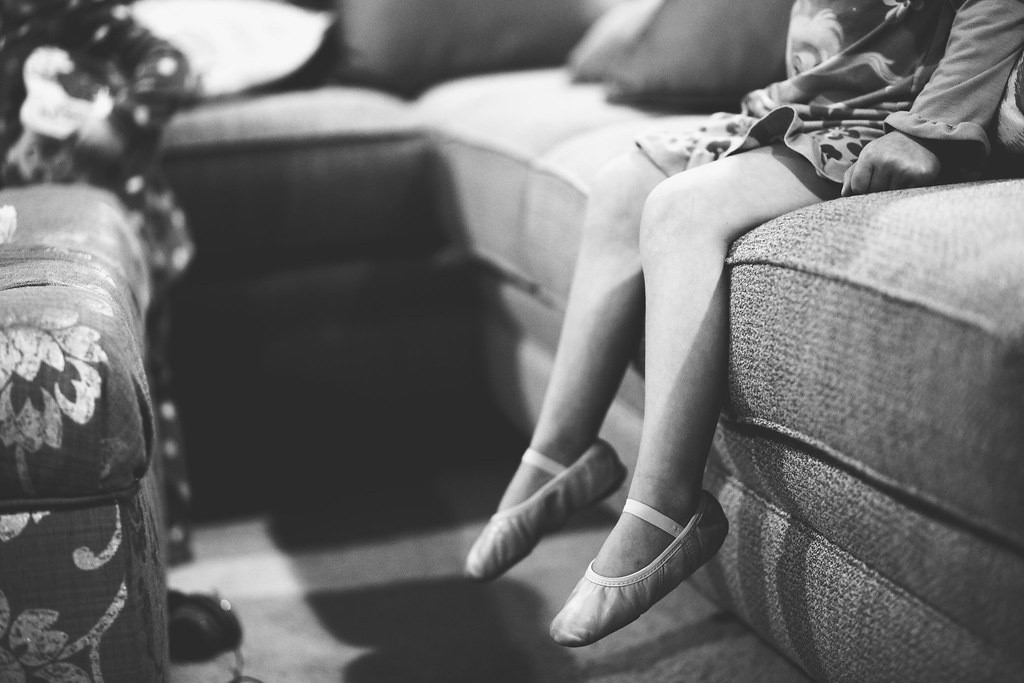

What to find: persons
left=464, top=0, right=1024, bottom=647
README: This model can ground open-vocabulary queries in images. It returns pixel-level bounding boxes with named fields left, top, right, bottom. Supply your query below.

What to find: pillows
left=567, top=1, right=796, bottom=90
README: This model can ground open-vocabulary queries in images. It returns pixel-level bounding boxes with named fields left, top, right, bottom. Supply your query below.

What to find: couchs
left=0, top=0, right=1023, bottom=683
